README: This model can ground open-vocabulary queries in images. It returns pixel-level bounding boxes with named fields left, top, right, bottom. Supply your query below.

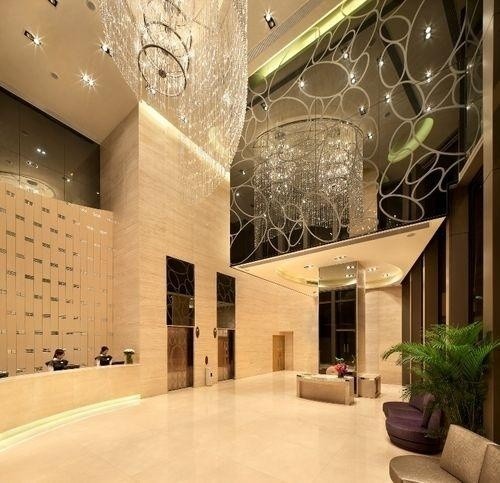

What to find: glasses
left=262, top=13, right=276, bottom=29
left=424, top=24, right=436, bottom=41
left=248, top=114, right=364, bottom=246
left=96, top=0, right=248, bottom=175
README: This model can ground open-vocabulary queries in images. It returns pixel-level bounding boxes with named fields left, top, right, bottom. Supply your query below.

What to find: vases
left=335, top=364, right=348, bottom=375
left=123, top=349, right=134, bottom=363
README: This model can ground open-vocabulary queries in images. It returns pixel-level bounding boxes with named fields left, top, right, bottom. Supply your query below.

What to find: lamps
left=389, top=425, right=499, bottom=483
left=383, top=388, right=445, bottom=454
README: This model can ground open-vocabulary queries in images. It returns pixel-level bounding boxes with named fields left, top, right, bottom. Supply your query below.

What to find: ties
left=112, top=361, right=125, bottom=364
left=0, top=373, right=9, bottom=378
left=64, top=364, right=80, bottom=369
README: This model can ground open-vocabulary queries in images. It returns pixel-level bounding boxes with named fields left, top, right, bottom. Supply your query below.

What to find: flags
left=338, top=373, right=343, bottom=378
left=126, top=358, right=132, bottom=363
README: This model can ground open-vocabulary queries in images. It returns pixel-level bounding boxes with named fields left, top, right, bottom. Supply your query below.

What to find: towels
left=205, top=367, right=218, bottom=386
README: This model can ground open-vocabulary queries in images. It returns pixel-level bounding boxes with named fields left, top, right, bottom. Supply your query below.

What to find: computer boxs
left=96, top=191, right=100, bottom=195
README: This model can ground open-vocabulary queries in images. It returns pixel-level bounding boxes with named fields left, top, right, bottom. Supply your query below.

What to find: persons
left=47, top=348, right=68, bottom=371
left=94, top=345, right=113, bottom=366
